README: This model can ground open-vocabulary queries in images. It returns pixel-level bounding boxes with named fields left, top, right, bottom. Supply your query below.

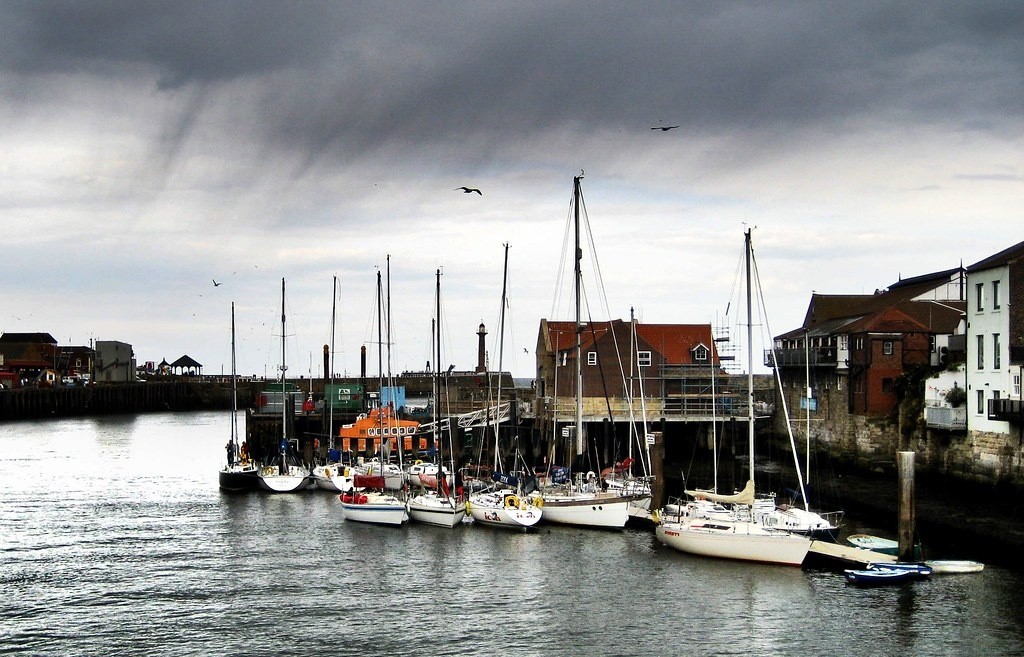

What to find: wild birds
left=523, top=347, right=528, bottom=353
left=455, top=187, right=482, bottom=195
left=213, top=279, right=222, bottom=287
left=651, top=126, right=678, bottom=130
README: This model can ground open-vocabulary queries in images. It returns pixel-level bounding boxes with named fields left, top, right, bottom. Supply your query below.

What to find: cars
left=65, top=378, right=90, bottom=388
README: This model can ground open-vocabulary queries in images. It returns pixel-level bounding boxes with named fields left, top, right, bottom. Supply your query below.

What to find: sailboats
left=218, top=168, right=846, bottom=568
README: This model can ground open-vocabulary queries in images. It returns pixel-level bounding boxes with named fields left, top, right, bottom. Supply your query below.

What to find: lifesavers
left=532, top=497, right=543, bottom=508
left=465, top=501, right=471, bottom=515
left=415, top=459, right=423, bottom=465
left=367, top=466, right=371, bottom=475
left=259, top=466, right=273, bottom=477
left=505, top=496, right=519, bottom=508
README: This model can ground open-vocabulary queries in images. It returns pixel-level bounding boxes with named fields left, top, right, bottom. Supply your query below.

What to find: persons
left=240, top=441, right=251, bottom=463
left=227, top=440, right=234, bottom=468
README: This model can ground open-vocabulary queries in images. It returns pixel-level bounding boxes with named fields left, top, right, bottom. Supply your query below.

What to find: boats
left=924, top=560, right=986, bottom=575
left=846, top=533, right=919, bottom=558
left=843, top=560, right=933, bottom=586
